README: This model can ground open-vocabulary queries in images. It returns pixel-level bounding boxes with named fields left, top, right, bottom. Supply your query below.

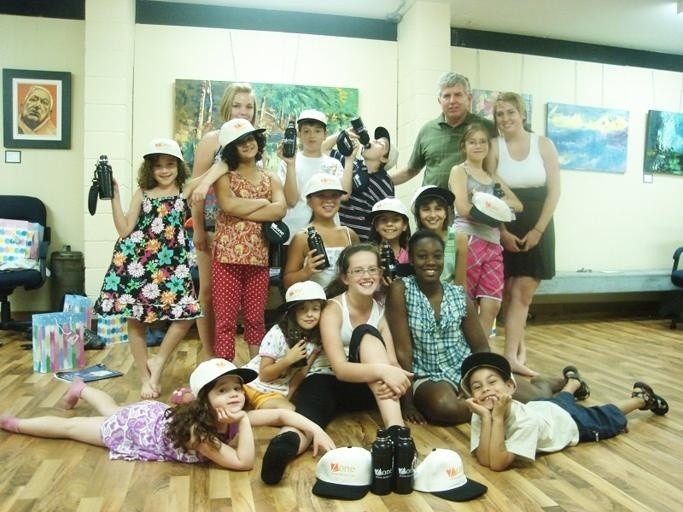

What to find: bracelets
left=534, top=227, right=544, bottom=234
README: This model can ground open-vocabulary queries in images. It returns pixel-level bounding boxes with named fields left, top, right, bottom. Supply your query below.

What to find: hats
left=469, top=193, right=512, bottom=228
left=296, top=110, right=328, bottom=126
left=312, top=445, right=372, bottom=501
left=220, top=118, right=266, bottom=146
left=189, top=357, right=259, bottom=400
left=277, top=281, right=327, bottom=311
left=373, top=126, right=399, bottom=171
left=365, top=196, right=410, bottom=222
left=410, top=185, right=456, bottom=214
left=142, top=139, right=184, bottom=161
left=304, top=173, right=348, bottom=198
left=411, top=448, right=489, bottom=502
left=460, top=351, right=518, bottom=399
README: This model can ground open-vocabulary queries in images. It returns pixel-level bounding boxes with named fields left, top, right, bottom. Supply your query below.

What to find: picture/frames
left=2, top=68, right=71, bottom=150
left=173, top=75, right=359, bottom=170
left=643, top=109, right=683, bottom=177
left=472, top=89, right=533, bottom=132
left=546, top=102, right=630, bottom=173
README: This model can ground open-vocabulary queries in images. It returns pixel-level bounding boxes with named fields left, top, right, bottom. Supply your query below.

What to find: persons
left=282, top=174, right=362, bottom=291
left=261, top=246, right=414, bottom=485
left=389, top=73, right=498, bottom=190
left=486, top=93, right=560, bottom=377
left=208, top=119, right=286, bottom=362
left=191, top=84, right=266, bottom=359
left=17, top=86, right=57, bottom=136
left=367, top=197, right=409, bottom=292
left=321, top=126, right=399, bottom=243
left=91, top=140, right=229, bottom=398
left=411, top=185, right=467, bottom=291
left=170, top=280, right=326, bottom=411
left=386, top=230, right=564, bottom=425
left=1, top=358, right=336, bottom=471
left=277, top=110, right=359, bottom=246
left=449, top=123, right=523, bottom=343
left=459, top=353, right=669, bottom=472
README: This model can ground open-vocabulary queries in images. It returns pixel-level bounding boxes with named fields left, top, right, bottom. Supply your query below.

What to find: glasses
left=348, top=266, right=381, bottom=275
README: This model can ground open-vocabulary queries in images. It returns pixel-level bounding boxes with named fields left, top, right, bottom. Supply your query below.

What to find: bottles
left=494, top=183, right=504, bottom=199
left=393, top=427, right=414, bottom=495
left=288, top=328, right=307, bottom=368
left=307, top=227, right=330, bottom=270
left=283, top=121, right=297, bottom=158
left=350, top=115, right=370, bottom=148
left=371, top=429, right=394, bottom=495
left=380, top=240, right=395, bottom=285
left=98, top=155, right=112, bottom=200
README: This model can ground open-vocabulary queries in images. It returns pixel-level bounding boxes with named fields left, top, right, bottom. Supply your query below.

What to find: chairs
left=671, top=248, right=683, bottom=329
left=0, top=194, right=52, bottom=335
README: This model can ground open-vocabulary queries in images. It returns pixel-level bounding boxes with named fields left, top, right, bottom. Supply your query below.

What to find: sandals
left=631, top=381, right=669, bottom=417
left=562, top=365, right=590, bottom=401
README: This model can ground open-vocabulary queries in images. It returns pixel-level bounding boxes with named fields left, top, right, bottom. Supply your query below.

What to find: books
left=54, top=364, right=123, bottom=384
